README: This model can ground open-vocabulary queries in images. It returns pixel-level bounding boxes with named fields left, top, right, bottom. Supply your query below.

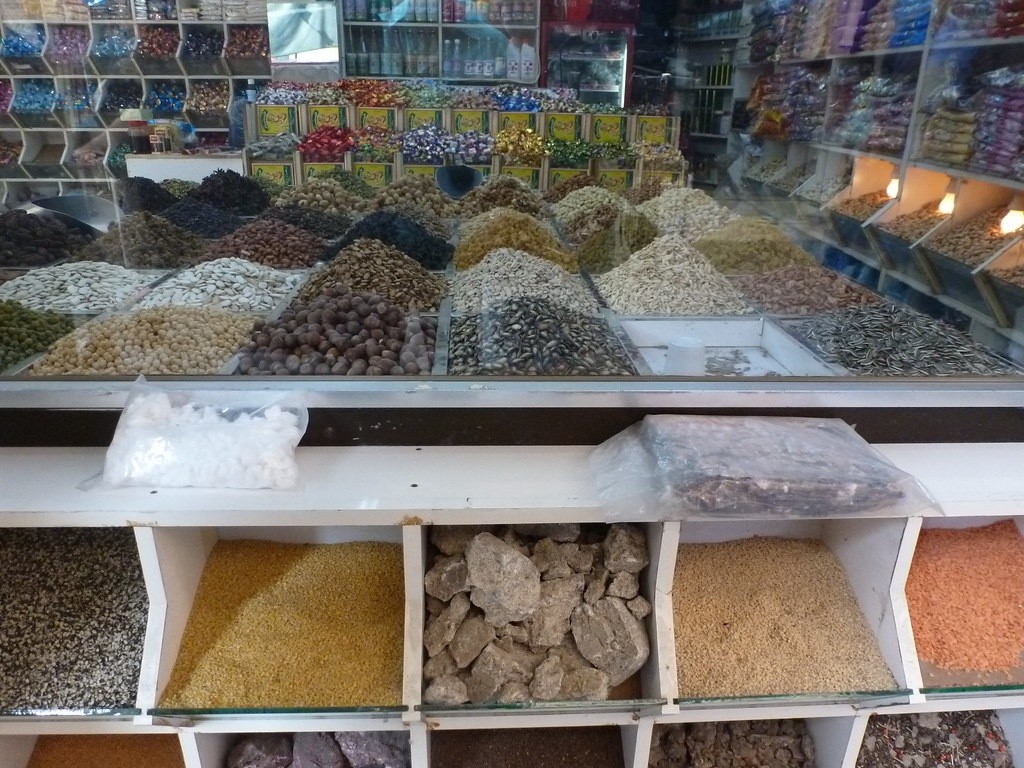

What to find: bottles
left=229, top=91, right=248, bottom=146
left=521, top=38, right=538, bottom=80
left=369, top=29, right=380, bottom=76
left=489, top=0, right=536, bottom=25
left=391, top=0, right=438, bottom=23
left=247, top=79, right=256, bottom=104
left=404, top=30, right=416, bottom=76
left=344, top=0, right=391, bottom=21
left=495, top=31, right=506, bottom=79
left=357, top=29, right=369, bottom=76
left=442, top=0, right=489, bottom=25
left=392, top=30, right=403, bottom=77
left=429, top=31, right=439, bottom=77
left=464, top=37, right=494, bottom=78
left=452, top=39, right=463, bottom=78
left=507, top=37, right=521, bottom=80
left=417, top=30, right=428, bottom=77
left=346, top=26, right=357, bottom=76
left=380, top=29, right=392, bottom=77
left=443, top=40, right=452, bottom=79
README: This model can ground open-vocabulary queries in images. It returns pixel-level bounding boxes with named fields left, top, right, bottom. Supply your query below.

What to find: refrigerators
left=540, top=21, right=634, bottom=110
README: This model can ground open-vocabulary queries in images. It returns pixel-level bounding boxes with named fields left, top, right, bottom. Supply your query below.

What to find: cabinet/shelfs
left=0, top=442, right=1024, bottom=768
left=680, top=0, right=1024, bottom=365
left=0, top=0, right=272, bottom=212
left=339, top=0, right=540, bottom=89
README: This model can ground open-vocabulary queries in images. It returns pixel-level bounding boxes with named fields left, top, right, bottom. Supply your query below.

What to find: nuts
left=0, top=233, right=448, bottom=312
left=238, top=285, right=438, bottom=377
left=442, top=231, right=1022, bottom=376
